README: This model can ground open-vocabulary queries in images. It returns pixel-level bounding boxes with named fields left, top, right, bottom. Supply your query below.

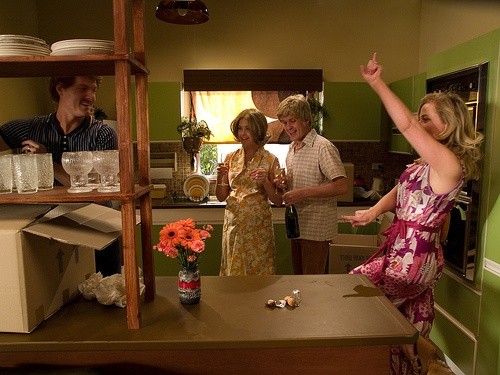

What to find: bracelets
left=269, top=191, right=278, bottom=200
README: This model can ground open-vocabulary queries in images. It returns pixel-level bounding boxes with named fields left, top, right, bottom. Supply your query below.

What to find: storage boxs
left=0, top=200, right=142, bottom=333
left=329, top=211, right=396, bottom=275
left=337, top=163, right=354, bottom=203
left=151, top=185, right=166, bottom=199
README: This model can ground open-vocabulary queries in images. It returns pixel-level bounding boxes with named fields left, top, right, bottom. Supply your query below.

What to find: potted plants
left=177, top=90, right=214, bottom=155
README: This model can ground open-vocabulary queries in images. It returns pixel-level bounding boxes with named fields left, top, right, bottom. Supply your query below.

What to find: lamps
left=155, top=0, right=210, bottom=25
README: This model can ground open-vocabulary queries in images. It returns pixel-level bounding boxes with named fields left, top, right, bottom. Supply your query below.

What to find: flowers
left=153, top=218, right=214, bottom=266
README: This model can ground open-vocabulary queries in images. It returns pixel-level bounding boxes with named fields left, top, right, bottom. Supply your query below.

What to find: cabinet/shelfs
left=131, top=82, right=183, bottom=143
left=0, top=0, right=156, bottom=329
left=321, top=28, right=500, bottom=375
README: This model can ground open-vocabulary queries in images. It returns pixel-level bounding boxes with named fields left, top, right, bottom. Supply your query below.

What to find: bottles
left=284, top=203, right=300, bottom=238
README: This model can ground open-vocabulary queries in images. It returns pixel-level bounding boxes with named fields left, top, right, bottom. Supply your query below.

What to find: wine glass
left=218, top=153, right=228, bottom=186
left=274, top=168, right=287, bottom=196
left=61, top=149, right=120, bottom=193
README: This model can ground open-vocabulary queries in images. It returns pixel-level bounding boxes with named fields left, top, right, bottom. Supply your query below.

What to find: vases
left=178, top=266, right=201, bottom=305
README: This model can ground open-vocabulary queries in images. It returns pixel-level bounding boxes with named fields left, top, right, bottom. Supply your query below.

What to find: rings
left=28, top=146, right=31, bottom=150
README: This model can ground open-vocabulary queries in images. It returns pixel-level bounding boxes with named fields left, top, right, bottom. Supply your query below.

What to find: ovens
left=425, top=63, right=488, bottom=132
left=441, top=177, right=474, bottom=281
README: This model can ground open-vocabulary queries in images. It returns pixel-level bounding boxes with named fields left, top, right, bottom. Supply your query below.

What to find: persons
left=216, top=109, right=283, bottom=276
left=273, top=94, right=348, bottom=274
left=0, top=73, right=121, bottom=279
left=341, top=52, right=483, bottom=375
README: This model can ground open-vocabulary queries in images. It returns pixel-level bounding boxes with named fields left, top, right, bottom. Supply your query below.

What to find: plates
left=0, top=34, right=52, bottom=57
left=188, top=185, right=205, bottom=202
left=49, top=38, right=117, bottom=55
left=183, top=174, right=209, bottom=200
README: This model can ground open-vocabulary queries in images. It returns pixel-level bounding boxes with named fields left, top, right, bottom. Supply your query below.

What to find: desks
left=0, top=274, right=419, bottom=375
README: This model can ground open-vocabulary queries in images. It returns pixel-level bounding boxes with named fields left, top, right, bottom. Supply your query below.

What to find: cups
left=14, top=153, right=38, bottom=194
left=0, top=153, right=13, bottom=194
left=36, top=153, right=54, bottom=190
left=10, top=154, right=18, bottom=192
